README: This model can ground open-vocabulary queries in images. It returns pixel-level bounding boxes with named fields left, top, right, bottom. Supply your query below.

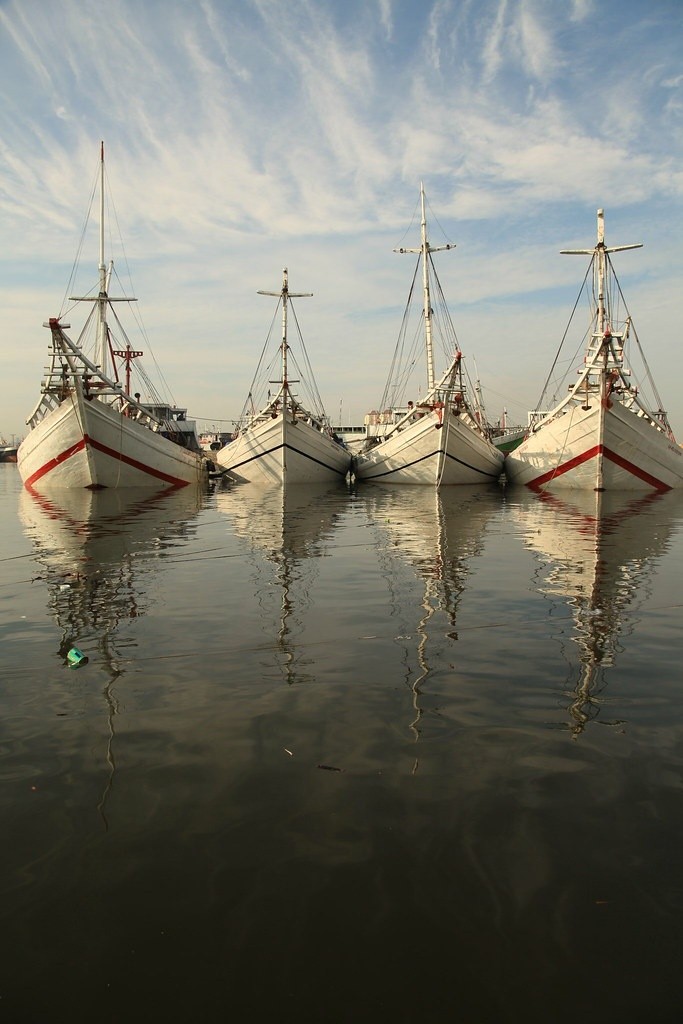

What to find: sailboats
left=351, top=181, right=510, bottom=488
left=501, top=207, right=682, bottom=495
left=212, top=264, right=356, bottom=487
left=14, top=135, right=209, bottom=495
